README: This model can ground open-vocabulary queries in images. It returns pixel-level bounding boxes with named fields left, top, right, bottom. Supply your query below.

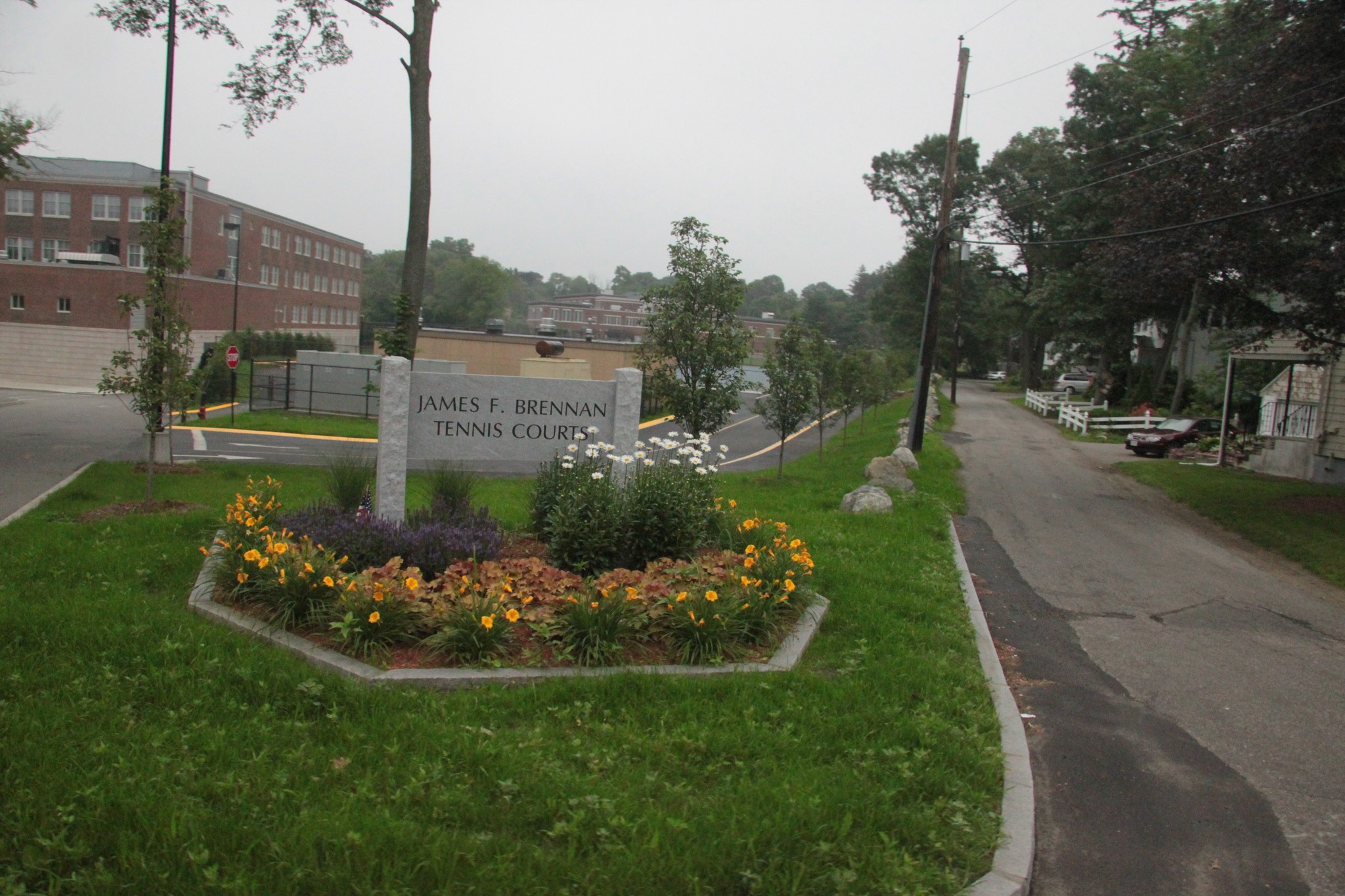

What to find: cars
left=1125, top=415, right=1247, bottom=456
left=987, top=371, right=1007, bottom=380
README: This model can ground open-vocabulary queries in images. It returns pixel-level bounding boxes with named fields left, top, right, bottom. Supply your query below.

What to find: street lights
left=223, top=221, right=242, bottom=426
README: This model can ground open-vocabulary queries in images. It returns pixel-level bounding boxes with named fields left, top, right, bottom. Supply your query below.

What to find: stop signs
left=226, top=345, right=240, bottom=369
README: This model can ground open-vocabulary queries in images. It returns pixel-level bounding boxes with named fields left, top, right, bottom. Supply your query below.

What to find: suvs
left=1054, top=372, right=1098, bottom=395
